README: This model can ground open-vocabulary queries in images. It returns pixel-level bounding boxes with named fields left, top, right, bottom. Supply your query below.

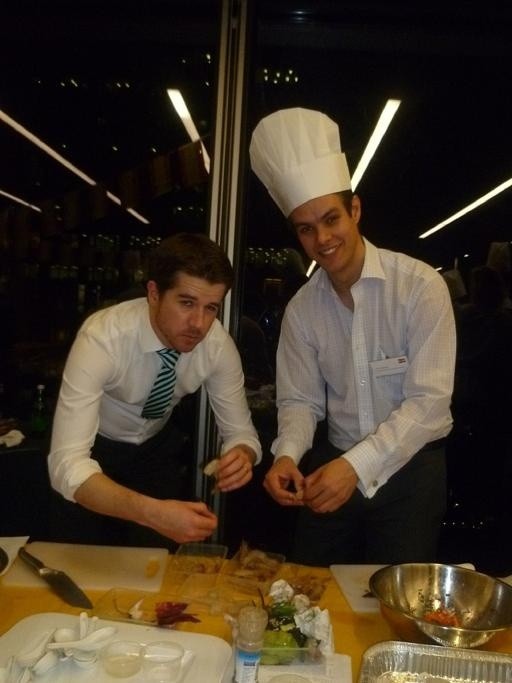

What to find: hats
left=485, top=241, right=512, bottom=270
left=249, top=105, right=354, bottom=219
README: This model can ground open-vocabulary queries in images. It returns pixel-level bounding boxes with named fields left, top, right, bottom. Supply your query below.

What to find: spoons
left=0, top=609, right=118, bottom=683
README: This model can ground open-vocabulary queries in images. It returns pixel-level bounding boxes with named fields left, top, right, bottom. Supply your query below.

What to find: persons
left=247, top=106, right=458, bottom=570
left=47, top=234, right=264, bottom=553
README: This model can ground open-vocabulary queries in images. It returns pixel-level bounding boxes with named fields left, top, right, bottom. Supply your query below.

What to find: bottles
left=233, top=606, right=269, bottom=682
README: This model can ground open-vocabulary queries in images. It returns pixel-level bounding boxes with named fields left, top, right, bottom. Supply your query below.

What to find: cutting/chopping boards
left=330, top=564, right=392, bottom=615
left=4, top=542, right=168, bottom=593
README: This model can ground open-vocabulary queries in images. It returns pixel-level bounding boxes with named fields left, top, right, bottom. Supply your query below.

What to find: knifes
left=18, top=546, right=95, bottom=612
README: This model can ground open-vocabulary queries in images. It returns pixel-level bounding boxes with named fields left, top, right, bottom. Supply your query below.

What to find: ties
left=140, top=346, right=182, bottom=419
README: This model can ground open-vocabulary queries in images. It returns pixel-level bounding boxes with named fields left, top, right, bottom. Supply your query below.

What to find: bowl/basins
left=100, top=640, right=141, bottom=677
left=368, top=562, right=511, bottom=649
left=141, top=640, right=184, bottom=681
